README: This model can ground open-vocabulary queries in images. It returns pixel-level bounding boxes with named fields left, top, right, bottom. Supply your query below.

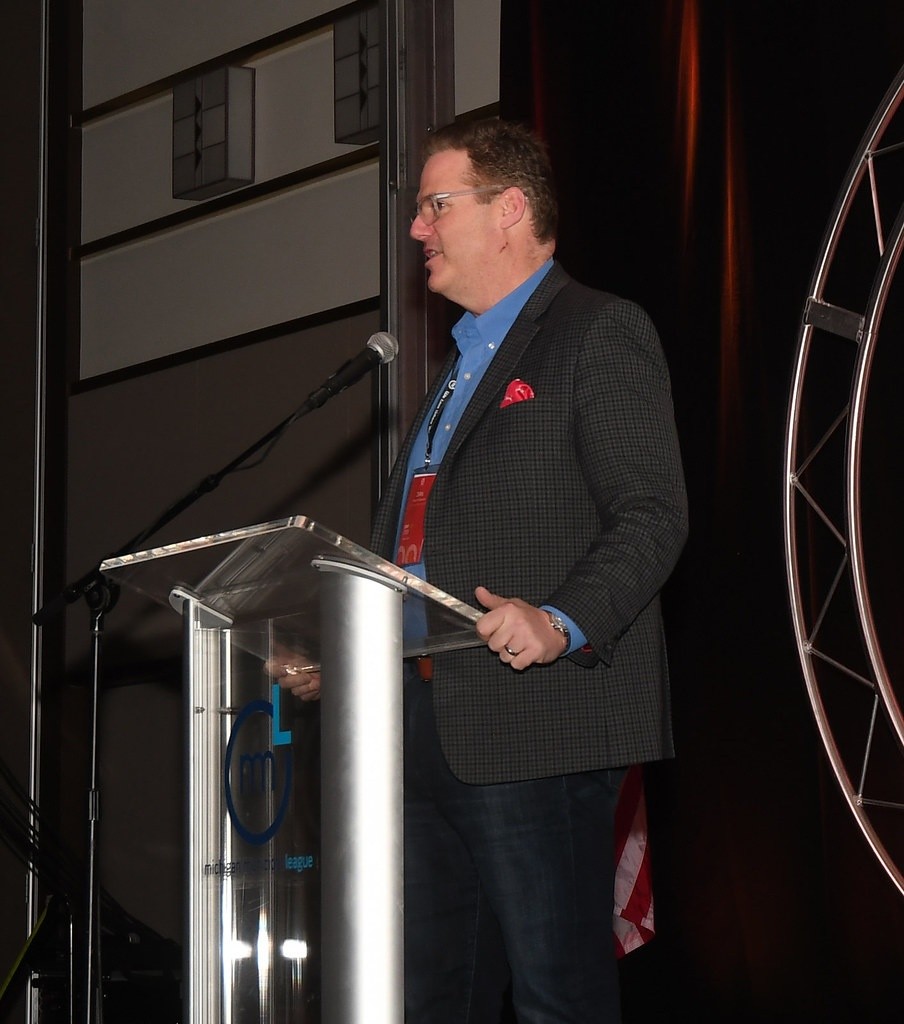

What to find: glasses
left=409, top=182, right=513, bottom=227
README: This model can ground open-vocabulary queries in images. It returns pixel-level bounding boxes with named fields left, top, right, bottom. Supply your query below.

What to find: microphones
left=294, top=332, right=400, bottom=418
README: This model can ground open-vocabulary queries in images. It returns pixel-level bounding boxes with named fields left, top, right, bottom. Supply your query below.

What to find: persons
left=504, top=644, right=519, bottom=657
left=261, top=109, right=694, bottom=1017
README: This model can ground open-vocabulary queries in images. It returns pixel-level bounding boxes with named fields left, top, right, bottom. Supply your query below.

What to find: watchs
left=548, top=611, right=571, bottom=655
